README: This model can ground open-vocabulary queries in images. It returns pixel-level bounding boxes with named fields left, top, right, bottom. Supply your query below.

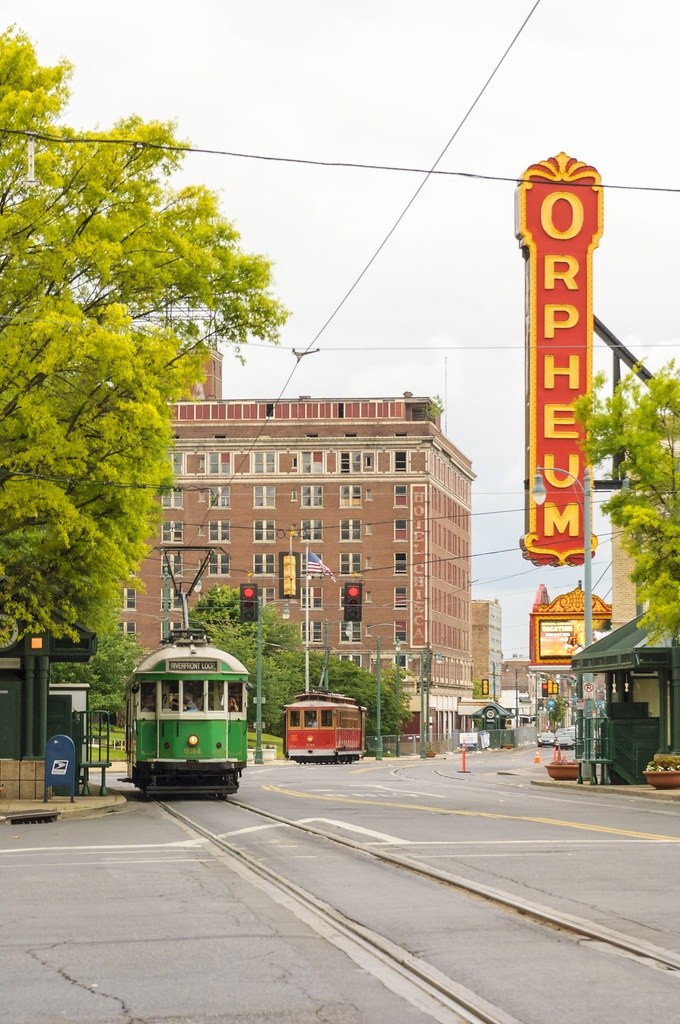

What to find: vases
left=544, top=764, right=580, bottom=781
left=642, top=771, right=680, bottom=790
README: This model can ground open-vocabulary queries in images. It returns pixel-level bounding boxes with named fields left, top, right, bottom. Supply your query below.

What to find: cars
left=538, top=725, right=576, bottom=750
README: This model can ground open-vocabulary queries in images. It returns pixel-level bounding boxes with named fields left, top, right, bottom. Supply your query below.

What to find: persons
left=567, top=634, right=584, bottom=654
left=143, top=686, right=163, bottom=712
left=172, top=696, right=202, bottom=710
left=308, top=718, right=317, bottom=726
left=229, top=695, right=239, bottom=712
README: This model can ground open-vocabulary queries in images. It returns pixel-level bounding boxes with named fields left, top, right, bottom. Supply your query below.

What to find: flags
left=308, top=550, right=336, bottom=583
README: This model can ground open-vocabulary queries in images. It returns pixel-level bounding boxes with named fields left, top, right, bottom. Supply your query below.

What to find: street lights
left=163, top=566, right=202, bottom=639
left=420, top=650, right=443, bottom=758
left=376, top=636, right=401, bottom=760
left=531, top=467, right=591, bottom=780
left=323, top=619, right=353, bottom=689
left=255, top=599, right=290, bottom=764
left=515, top=667, right=531, bottom=727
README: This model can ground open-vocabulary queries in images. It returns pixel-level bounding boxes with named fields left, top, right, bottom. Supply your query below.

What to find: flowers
left=550, top=756, right=577, bottom=765
left=646, top=752, right=680, bottom=772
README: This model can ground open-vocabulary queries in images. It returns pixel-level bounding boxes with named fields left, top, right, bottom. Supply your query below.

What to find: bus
left=125, top=630, right=250, bottom=802
left=283, top=687, right=367, bottom=765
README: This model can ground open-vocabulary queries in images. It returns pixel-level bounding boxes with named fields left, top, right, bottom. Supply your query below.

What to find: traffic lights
left=344, top=583, right=362, bottom=621
left=542, top=681, right=548, bottom=698
left=240, top=583, right=258, bottom=621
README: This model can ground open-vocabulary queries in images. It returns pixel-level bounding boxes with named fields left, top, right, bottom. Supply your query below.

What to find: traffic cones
left=533, top=749, right=542, bottom=765
